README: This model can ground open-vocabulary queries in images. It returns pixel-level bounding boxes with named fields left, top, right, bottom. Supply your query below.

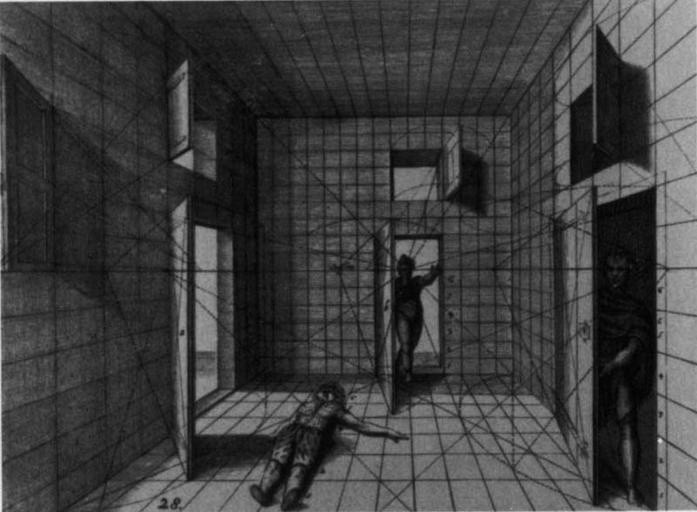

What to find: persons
left=249, top=381, right=409, bottom=510
left=594, top=244, right=651, bottom=505
left=397, top=253, right=440, bottom=382
